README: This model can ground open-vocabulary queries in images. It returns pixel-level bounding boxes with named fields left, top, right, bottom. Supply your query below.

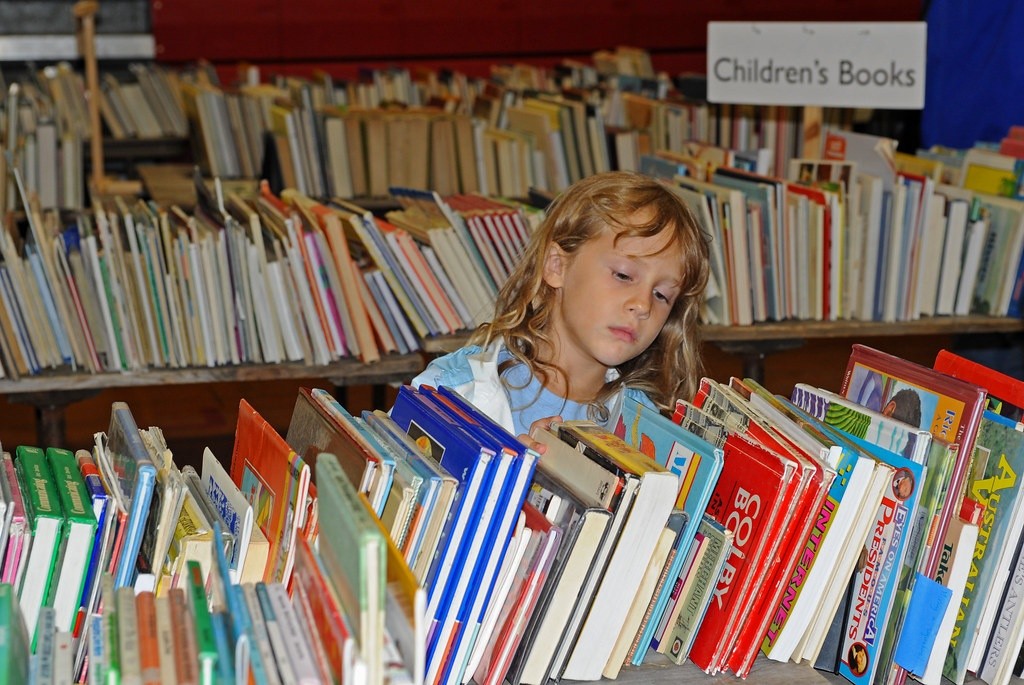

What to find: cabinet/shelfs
left=688, top=314, right=1022, bottom=394
left=2, top=353, right=427, bottom=463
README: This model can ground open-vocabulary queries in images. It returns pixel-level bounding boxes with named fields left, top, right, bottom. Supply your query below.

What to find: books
left=1, top=0, right=1023, bottom=375
left=3, top=342, right=1024, bottom=685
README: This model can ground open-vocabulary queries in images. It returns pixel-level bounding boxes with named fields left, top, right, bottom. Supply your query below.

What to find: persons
left=356, top=165, right=714, bottom=439
